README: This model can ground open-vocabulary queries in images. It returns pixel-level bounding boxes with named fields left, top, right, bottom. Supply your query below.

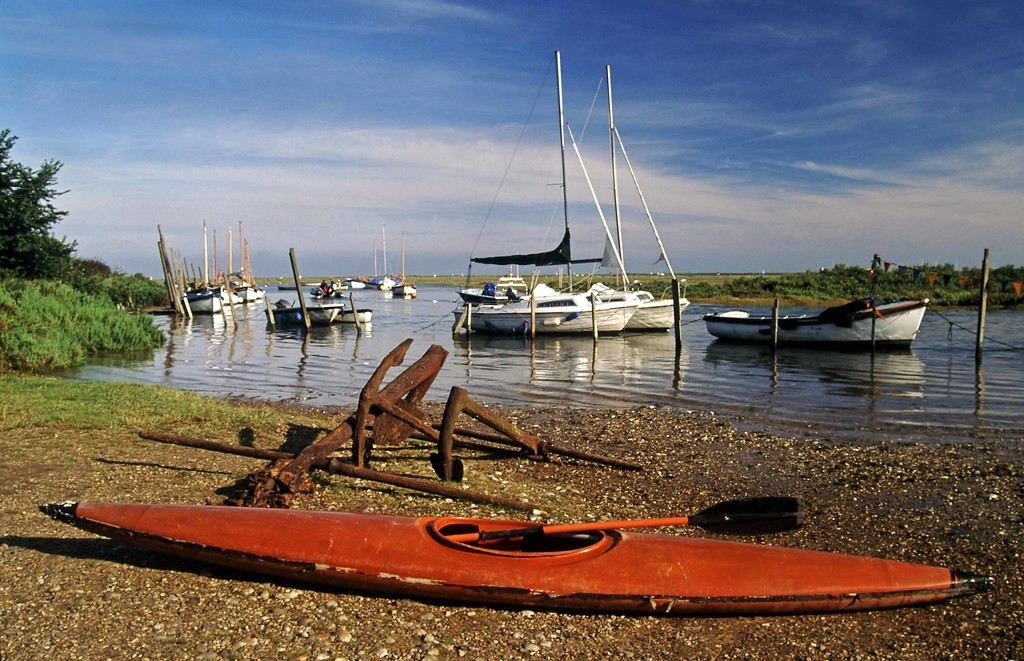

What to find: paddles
left=445, top=495, right=809, bottom=545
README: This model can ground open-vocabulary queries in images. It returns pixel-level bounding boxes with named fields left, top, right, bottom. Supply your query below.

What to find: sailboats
left=365, top=225, right=403, bottom=291
left=172, top=218, right=265, bottom=313
left=391, top=233, right=418, bottom=299
left=452, top=51, right=691, bottom=334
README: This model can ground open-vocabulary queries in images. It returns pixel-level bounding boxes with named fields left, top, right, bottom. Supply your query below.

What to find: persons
left=485, top=283, right=495, bottom=296
left=320, top=279, right=328, bottom=293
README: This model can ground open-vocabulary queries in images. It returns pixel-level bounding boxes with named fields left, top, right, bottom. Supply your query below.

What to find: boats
left=264, top=303, right=346, bottom=324
left=336, top=309, right=373, bottom=323
left=340, top=278, right=365, bottom=290
left=702, top=297, right=929, bottom=348
left=36, top=495, right=998, bottom=616
